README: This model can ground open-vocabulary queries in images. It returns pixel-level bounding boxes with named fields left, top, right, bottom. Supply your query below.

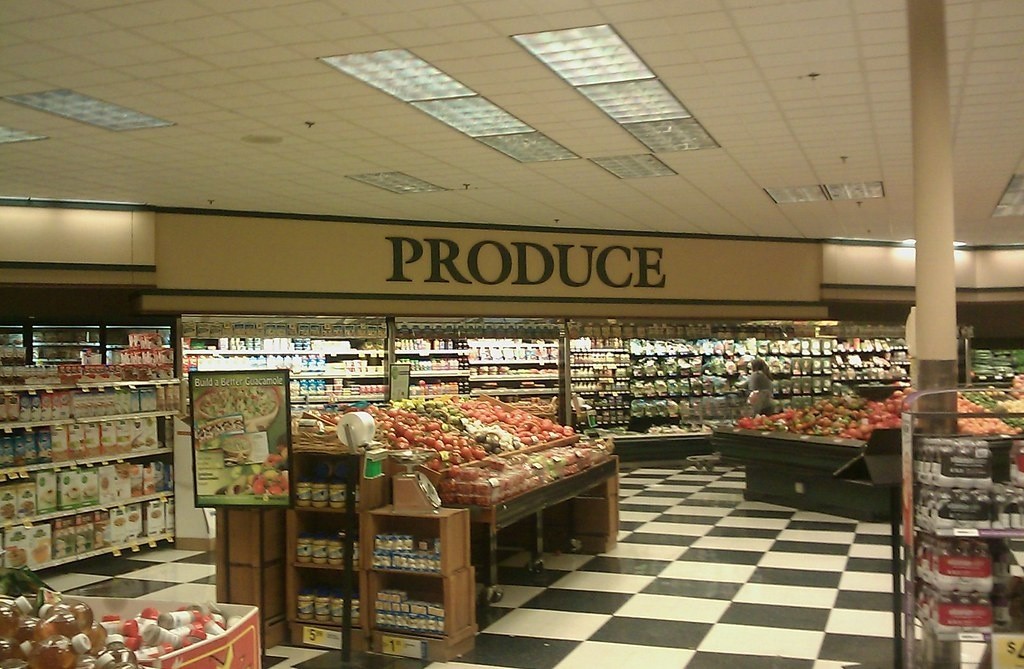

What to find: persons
left=735, top=358, right=775, bottom=417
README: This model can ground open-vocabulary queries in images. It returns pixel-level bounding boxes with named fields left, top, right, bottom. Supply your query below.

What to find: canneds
left=296, top=481, right=359, bottom=623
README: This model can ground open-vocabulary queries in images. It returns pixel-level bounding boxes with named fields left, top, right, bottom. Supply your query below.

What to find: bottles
left=913, top=437, right=992, bottom=486
left=1009, top=438, right=1024, bottom=486
left=915, top=487, right=1024, bottom=531
left=916, top=582, right=994, bottom=641
left=916, top=536, right=994, bottom=592
left=0, top=594, right=144, bottom=669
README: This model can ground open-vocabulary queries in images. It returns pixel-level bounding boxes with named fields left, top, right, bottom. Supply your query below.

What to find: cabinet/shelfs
left=891, top=380, right=1024, bottom=669
left=0, top=319, right=190, bottom=572
left=284, top=394, right=620, bottom=659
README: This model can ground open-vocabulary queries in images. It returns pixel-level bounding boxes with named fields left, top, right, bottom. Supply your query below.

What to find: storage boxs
left=286, top=564, right=368, bottom=626
left=370, top=627, right=477, bottom=662
left=573, top=497, right=618, bottom=536
left=286, top=508, right=368, bottom=570
left=585, top=473, right=620, bottom=498
left=0, top=437, right=13, bottom=467
left=0, top=498, right=174, bottom=569
left=14, top=437, right=24, bottom=466
left=80, top=467, right=99, bottom=507
left=369, top=571, right=477, bottom=631
left=98, top=459, right=173, bottom=498
left=16, top=482, right=36, bottom=519
left=287, top=621, right=368, bottom=653
left=573, top=534, right=621, bottom=553
left=60, top=596, right=261, bottom=669
left=366, top=506, right=473, bottom=576
left=290, top=449, right=388, bottom=508
left=26, top=471, right=57, bottom=515
left=57, top=468, right=80, bottom=509
left=0, top=387, right=180, bottom=457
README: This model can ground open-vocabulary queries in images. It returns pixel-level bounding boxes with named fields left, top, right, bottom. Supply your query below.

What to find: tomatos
left=253, top=477, right=289, bottom=495
left=322, top=407, right=486, bottom=471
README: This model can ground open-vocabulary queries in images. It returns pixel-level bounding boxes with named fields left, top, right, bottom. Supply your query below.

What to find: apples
left=843, top=394, right=908, bottom=440
